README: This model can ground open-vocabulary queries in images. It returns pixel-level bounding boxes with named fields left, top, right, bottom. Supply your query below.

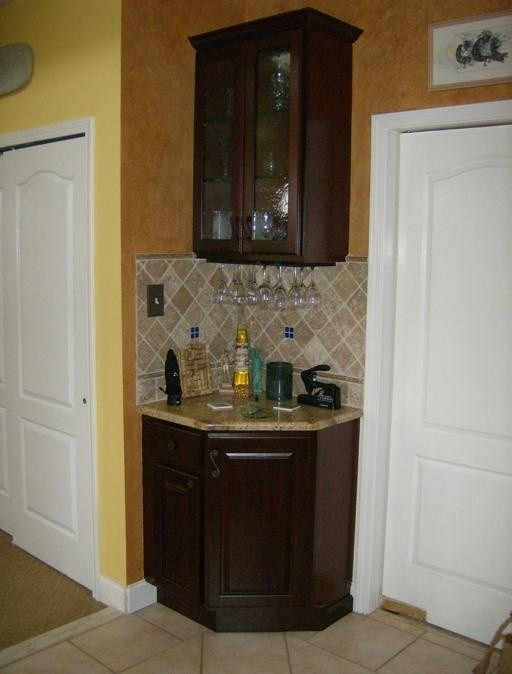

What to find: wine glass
left=212, top=262, right=320, bottom=311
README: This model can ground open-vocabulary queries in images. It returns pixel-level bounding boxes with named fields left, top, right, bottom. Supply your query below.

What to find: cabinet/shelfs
left=141, top=412, right=361, bottom=632
left=187, top=6, right=364, bottom=267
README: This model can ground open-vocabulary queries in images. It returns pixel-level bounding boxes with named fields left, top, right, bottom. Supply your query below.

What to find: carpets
left=0, top=528, right=124, bottom=668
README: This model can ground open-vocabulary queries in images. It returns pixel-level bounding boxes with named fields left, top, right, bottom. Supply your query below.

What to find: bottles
left=265, top=58, right=287, bottom=111
left=222, top=324, right=262, bottom=393
